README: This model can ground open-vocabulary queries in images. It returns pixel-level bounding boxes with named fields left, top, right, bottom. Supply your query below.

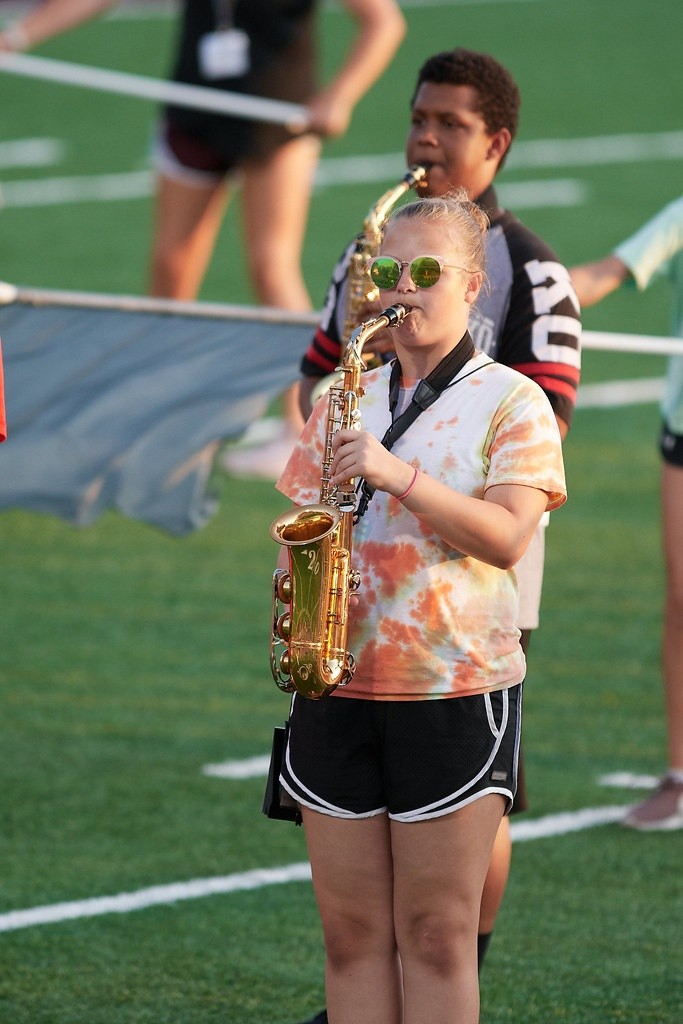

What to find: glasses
left=369, top=258, right=475, bottom=290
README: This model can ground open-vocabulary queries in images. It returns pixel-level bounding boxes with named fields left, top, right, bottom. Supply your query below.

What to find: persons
left=0, top=1, right=406, bottom=486
left=292, top=47, right=586, bottom=1024
left=258, top=185, right=565, bottom=1024
left=526, top=185, right=683, bottom=846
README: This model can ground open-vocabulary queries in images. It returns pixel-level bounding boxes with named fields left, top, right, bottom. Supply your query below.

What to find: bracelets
left=5, top=14, right=34, bottom=60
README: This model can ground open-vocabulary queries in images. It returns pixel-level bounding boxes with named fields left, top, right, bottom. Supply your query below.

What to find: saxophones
left=309, top=150, right=431, bottom=411
left=269, top=301, right=411, bottom=702
